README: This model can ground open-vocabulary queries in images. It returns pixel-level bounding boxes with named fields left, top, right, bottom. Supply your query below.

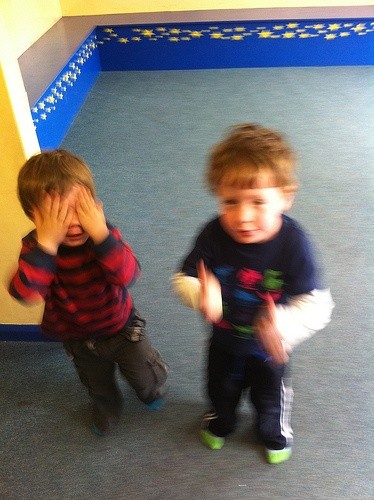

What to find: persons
left=7, top=149, right=168, bottom=440
left=167, top=122, right=335, bottom=465
